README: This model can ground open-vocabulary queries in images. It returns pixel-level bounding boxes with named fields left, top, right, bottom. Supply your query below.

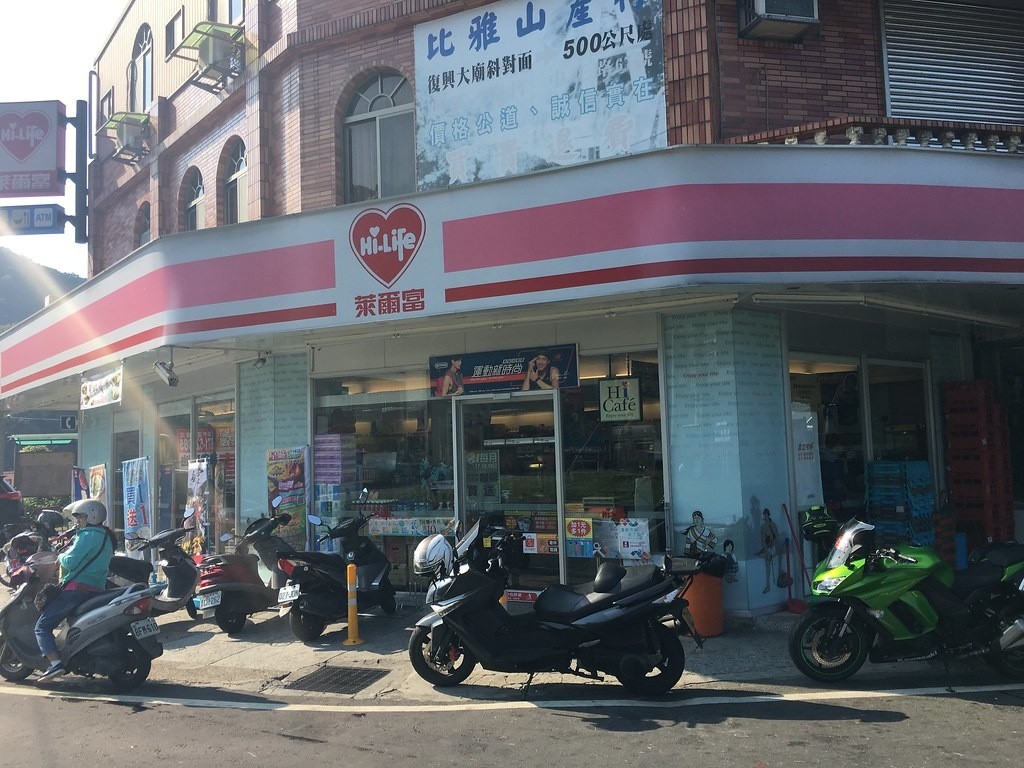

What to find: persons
left=821, top=433, right=844, bottom=503
left=440, top=355, right=465, bottom=397
left=31, top=499, right=118, bottom=684
left=521, top=348, right=559, bottom=390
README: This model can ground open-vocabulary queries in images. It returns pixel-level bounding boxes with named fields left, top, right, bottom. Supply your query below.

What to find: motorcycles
left=788, top=512, right=1024, bottom=684
left=103, top=506, right=198, bottom=622
left=277, top=487, right=398, bottom=644
left=406, top=517, right=706, bottom=708
left=0, top=536, right=165, bottom=691
left=196, top=492, right=295, bottom=634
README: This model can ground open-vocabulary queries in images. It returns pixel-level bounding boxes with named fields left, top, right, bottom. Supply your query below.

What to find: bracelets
left=535, top=378, right=540, bottom=382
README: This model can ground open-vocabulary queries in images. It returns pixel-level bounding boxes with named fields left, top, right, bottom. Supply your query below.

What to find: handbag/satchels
left=34, top=582, right=61, bottom=612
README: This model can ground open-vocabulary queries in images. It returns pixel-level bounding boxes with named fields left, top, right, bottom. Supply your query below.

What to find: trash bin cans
left=673, top=558, right=728, bottom=636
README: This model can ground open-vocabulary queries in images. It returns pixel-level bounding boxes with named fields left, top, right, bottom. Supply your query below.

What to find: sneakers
left=38, top=663, right=66, bottom=682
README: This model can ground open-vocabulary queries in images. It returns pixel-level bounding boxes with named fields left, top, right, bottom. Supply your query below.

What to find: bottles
left=351, top=499, right=424, bottom=517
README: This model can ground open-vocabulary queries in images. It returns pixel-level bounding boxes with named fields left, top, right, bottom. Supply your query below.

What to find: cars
left=0, top=476, right=24, bottom=548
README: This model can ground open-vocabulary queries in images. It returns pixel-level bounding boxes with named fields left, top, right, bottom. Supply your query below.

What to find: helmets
left=63, top=499, right=107, bottom=525
left=413, top=534, right=454, bottom=580
